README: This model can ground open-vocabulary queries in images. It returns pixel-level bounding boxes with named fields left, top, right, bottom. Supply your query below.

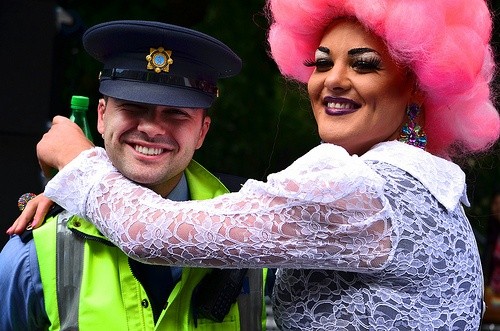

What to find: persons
left=5, top=1, right=499, bottom=331
left=0, top=17, right=274, bottom=331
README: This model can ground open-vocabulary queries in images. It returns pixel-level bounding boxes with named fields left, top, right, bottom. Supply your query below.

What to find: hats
left=82, top=18, right=243, bottom=108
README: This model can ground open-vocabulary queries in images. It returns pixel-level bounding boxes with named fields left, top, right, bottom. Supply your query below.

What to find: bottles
left=68, top=95, right=93, bottom=143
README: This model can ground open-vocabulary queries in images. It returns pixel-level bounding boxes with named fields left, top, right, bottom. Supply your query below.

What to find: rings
left=18, top=193, right=38, bottom=212
